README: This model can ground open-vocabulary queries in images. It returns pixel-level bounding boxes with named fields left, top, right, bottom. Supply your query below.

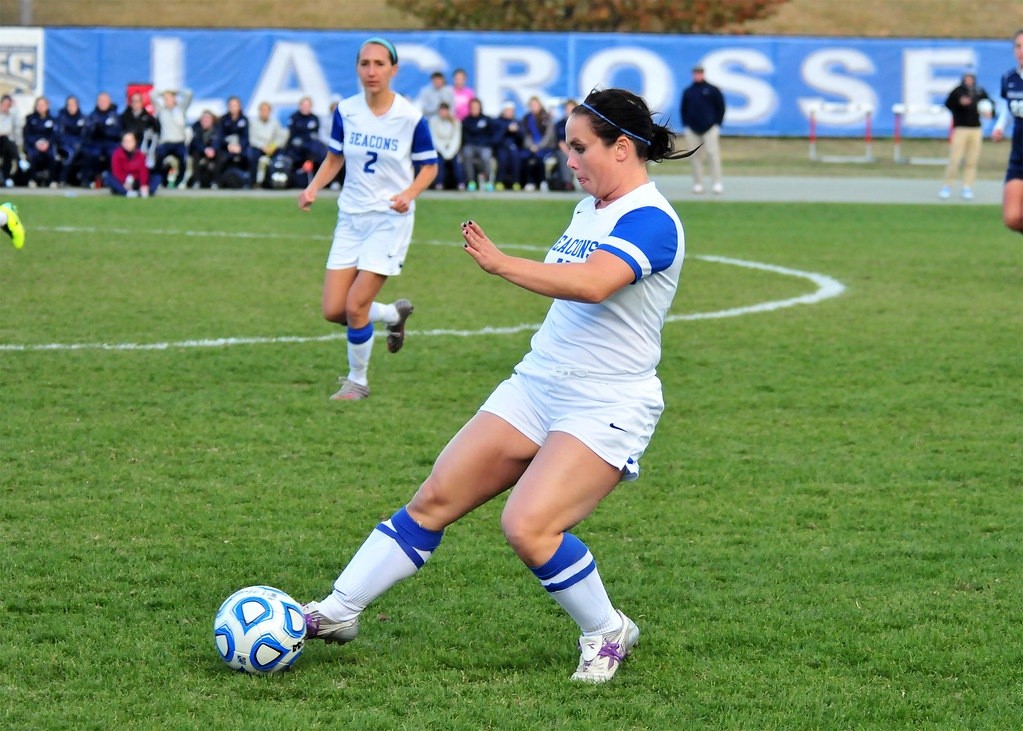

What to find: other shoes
left=939, top=187, right=950, bottom=198
left=962, top=186, right=974, bottom=200
left=692, top=183, right=704, bottom=194
left=711, top=183, right=723, bottom=193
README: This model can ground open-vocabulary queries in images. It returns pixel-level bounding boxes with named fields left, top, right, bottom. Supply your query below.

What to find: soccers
left=213, top=583, right=309, bottom=674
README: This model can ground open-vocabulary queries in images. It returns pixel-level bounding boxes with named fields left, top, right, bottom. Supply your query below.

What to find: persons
left=414, top=70, right=580, bottom=191
left=992, top=30, right=1023, bottom=232
left=102, top=131, right=162, bottom=198
left=299, top=38, right=438, bottom=402
left=303, top=89, right=685, bottom=684
left=680, top=67, right=725, bottom=193
left=0, top=201, right=25, bottom=249
left=0, top=88, right=345, bottom=189
left=938, top=62, right=995, bottom=199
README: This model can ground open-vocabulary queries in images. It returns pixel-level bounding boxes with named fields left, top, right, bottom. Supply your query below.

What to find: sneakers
left=301, top=601, right=357, bottom=647
left=386, top=299, right=412, bottom=352
left=328, top=376, right=368, bottom=399
left=0, top=201, right=24, bottom=248
left=571, top=607, right=639, bottom=683
